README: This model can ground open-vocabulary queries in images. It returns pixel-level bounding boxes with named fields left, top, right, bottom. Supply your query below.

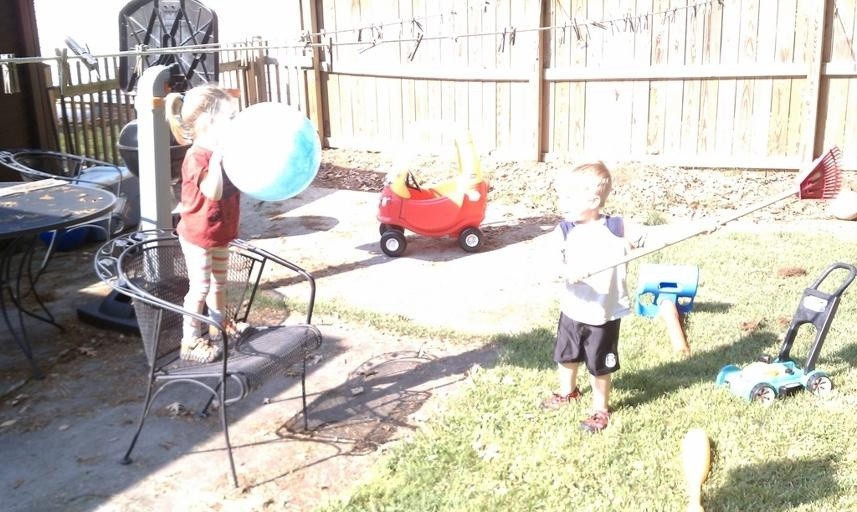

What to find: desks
left=0, top=182, right=117, bottom=380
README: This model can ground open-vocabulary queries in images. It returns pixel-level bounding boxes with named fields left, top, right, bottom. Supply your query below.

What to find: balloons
left=219, top=100, right=323, bottom=206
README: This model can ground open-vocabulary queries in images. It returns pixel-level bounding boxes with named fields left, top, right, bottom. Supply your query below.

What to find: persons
left=160, top=82, right=251, bottom=364
left=540, top=157, right=728, bottom=439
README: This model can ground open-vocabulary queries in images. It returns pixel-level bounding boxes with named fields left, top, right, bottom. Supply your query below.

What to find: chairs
left=94, top=227, right=323, bottom=487
left=0, top=148, right=129, bottom=321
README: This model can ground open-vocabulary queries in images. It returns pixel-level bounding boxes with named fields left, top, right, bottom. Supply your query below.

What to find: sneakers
left=209, top=319, right=251, bottom=339
left=581, top=411, right=610, bottom=433
left=539, top=388, right=581, bottom=412
left=179, top=338, right=222, bottom=362
